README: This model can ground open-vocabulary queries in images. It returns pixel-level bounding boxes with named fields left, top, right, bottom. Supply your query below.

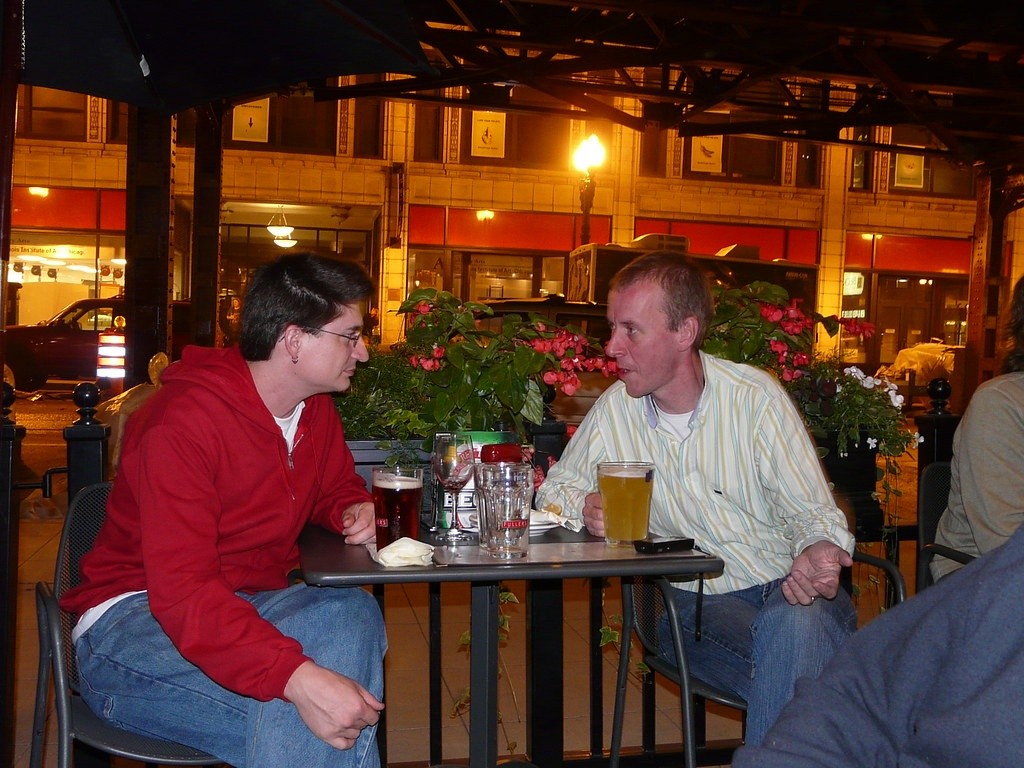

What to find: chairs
left=607, top=547, right=909, bottom=768
left=29, top=482, right=230, bottom=768
left=915, top=462, right=980, bottom=595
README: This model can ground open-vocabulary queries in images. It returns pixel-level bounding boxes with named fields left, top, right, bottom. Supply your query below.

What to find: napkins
left=364, top=536, right=437, bottom=568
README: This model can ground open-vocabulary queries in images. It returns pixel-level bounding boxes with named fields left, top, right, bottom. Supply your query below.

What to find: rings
left=811, top=596, right=815, bottom=601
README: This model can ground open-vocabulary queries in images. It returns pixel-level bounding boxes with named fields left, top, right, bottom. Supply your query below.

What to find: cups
left=596, top=461, right=656, bottom=550
left=474, top=461, right=535, bottom=560
left=371, top=465, right=423, bottom=551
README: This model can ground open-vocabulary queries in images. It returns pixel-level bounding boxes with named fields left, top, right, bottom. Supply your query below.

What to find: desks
left=298, top=521, right=724, bottom=768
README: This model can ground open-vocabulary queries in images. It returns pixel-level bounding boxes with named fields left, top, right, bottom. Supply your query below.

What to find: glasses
left=279, top=323, right=362, bottom=349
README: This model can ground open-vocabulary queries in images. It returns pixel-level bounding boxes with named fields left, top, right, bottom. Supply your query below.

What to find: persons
left=731, top=518, right=1024, bottom=768
left=929, top=275, right=1024, bottom=581
left=534, top=249, right=859, bottom=748
left=60, top=254, right=390, bottom=768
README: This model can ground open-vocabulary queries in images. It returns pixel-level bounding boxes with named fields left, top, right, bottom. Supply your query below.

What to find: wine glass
left=432, top=433, right=475, bottom=541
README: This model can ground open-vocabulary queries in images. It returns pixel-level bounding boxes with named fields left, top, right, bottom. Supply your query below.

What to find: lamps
left=266, top=204, right=298, bottom=249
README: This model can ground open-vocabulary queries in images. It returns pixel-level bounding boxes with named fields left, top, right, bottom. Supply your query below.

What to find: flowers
left=700, top=282, right=925, bottom=615
left=368, top=289, right=624, bottom=493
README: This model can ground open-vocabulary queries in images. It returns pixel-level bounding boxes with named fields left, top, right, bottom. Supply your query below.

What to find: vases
left=808, top=428, right=884, bottom=530
left=432, top=431, right=515, bottom=532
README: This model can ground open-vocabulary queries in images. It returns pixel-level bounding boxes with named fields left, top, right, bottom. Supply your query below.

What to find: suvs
left=2, top=296, right=190, bottom=396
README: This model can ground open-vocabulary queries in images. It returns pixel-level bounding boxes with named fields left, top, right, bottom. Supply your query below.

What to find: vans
left=432, top=292, right=613, bottom=359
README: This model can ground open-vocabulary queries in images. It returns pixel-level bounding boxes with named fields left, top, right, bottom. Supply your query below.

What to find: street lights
left=566, top=134, right=609, bottom=247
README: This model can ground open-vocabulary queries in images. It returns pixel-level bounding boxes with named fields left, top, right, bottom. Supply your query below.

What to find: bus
left=565, top=232, right=969, bottom=370
